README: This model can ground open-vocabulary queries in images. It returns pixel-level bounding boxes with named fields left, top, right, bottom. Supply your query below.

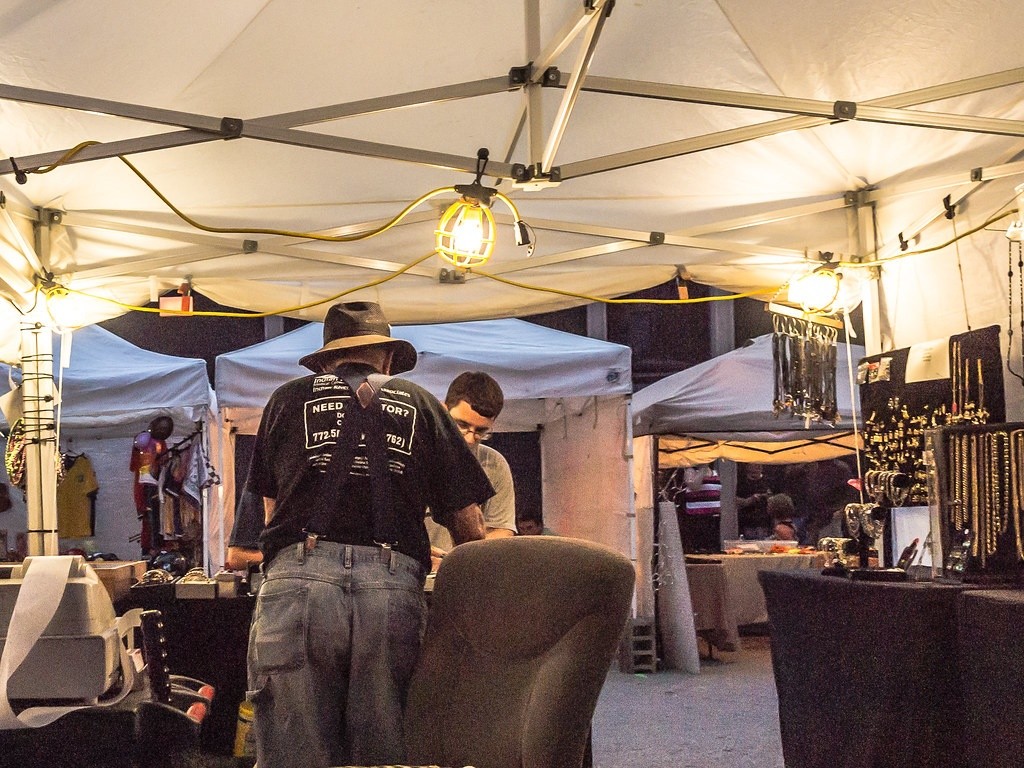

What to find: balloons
left=133, top=431, right=166, bottom=478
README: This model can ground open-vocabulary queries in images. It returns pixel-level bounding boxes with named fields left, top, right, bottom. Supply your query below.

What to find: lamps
left=62, top=448, right=88, bottom=460
left=432, top=182, right=498, bottom=269
left=787, top=258, right=861, bottom=317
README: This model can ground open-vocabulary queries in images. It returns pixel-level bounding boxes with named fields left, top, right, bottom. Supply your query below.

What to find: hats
left=299, top=301, right=417, bottom=376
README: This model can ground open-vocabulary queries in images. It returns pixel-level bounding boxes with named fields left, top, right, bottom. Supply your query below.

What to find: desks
left=684, top=549, right=860, bottom=637
left=650, top=564, right=741, bottom=662
left=755, top=567, right=1024, bottom=768
left=952, top=588, right=1024, bottom=768
left=122, top=595, right=256, bottom=758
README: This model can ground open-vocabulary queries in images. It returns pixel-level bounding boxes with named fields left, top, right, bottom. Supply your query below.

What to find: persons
left=686, top=459, right=856, bottom=555
left=225, top=481, right=266, bottom=573
left=247, top=301, right=497, bottom=768
left=424, top=371, right=519, bottom=575
left=515, top=503, right=561, bottom=536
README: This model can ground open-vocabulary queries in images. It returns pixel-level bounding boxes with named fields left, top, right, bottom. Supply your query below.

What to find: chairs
left=400, top=535, right=635, bottom=768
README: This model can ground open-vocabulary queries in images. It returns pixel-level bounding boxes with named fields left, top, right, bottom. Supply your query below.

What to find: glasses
left=449, top=412, right=494, bottom=441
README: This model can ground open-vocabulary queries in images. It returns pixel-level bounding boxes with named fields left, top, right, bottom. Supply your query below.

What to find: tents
left=632, top=328, right=865, bottom=672
left=0, top=318, right=631, bottom=672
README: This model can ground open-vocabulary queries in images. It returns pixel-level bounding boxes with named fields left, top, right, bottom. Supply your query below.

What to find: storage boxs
left=90, top=561, right=133, bottom=604
left=174, top=577, right=218, bottom=600
left=103, top=560, right=146, bottom=579
left=130, top=575, right=181, bottom=601
